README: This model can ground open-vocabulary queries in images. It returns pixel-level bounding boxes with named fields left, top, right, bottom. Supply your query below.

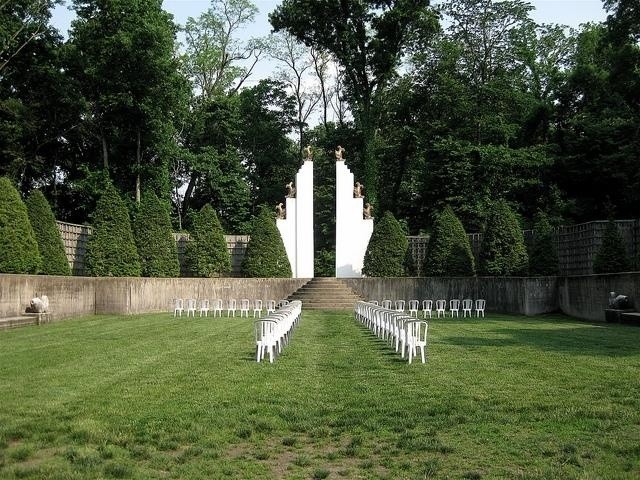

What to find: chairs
left=355, top=298, right=487, bottom=365
left=171, top=298, right=303, bottom=363
